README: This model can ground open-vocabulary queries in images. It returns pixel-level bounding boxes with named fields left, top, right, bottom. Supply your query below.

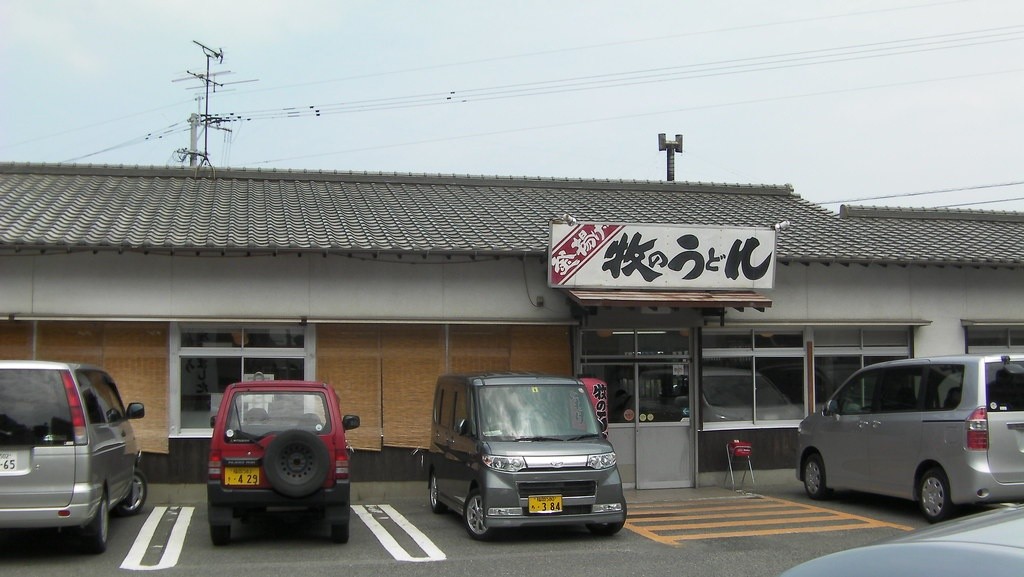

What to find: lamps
left=771, top=220, right=791, bottom=232
left=552, top=214, right=576, bottom=226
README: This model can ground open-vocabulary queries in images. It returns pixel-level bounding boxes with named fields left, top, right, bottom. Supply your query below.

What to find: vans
left=795, top=353, right=1024, bottom=525
left=428, top=371, right=627, bottom=541
left=640, top=366, right=805, bottom=422
left=0, top=358, right=148, bottom=556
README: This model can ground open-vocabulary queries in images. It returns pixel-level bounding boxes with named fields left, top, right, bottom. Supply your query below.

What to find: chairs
left=244, top=408, right=268, bottom=423
left=296, top=413, right=321, bottom=430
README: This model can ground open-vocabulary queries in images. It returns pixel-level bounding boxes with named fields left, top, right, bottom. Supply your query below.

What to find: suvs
left=207, top=380, right=361, bottom=547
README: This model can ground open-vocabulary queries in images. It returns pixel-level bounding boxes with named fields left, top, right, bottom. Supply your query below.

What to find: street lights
left=658, top=132, right=682, bottom=182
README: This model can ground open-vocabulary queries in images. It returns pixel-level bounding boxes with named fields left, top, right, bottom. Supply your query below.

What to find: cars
left=781, top=501, right=1024, bottom=576
